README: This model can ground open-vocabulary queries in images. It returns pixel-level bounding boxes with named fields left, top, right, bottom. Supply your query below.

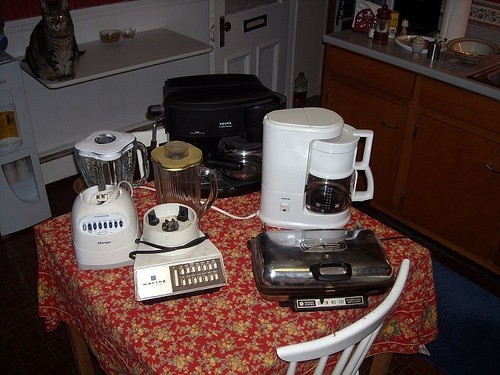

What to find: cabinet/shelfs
left=398, top=74, right=498, bottom=275
left=319, top=42, right=416, bottom=213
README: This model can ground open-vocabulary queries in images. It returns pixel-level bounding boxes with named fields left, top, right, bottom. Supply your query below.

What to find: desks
left=32, top=179, right=431, bottom=374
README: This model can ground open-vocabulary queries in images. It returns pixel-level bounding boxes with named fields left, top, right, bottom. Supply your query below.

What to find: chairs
left=276, top=258, right=410, bottom=369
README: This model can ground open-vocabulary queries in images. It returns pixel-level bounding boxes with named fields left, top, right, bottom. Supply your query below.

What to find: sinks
left=467, top=60, right=492, bottom=90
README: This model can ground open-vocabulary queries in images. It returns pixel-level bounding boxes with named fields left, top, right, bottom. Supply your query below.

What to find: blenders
left=73, top=130, right=149, bottom=270
left=133, top=141, right=228, bottom=305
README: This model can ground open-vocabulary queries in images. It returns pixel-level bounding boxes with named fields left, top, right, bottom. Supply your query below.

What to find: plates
left=395, top=35, right=448, bottom=54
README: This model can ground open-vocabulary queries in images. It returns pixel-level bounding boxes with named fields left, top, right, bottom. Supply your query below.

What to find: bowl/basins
left=100, top=30, right=120, bottom=41
left=119, top=24, right=136, bottom=37
left=445, top=37, right=499, bottom=60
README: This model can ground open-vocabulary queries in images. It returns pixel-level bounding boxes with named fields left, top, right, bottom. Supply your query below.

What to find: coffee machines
left=146, top=74, right=287, bottom=199
left=260, top=108, right=375, bottom=229
